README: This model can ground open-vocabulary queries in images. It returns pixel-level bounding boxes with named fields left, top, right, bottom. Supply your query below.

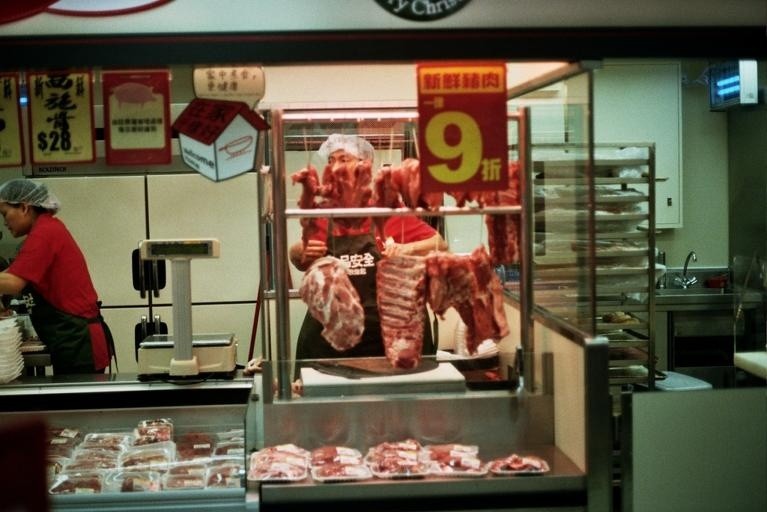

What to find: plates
left=245, top=449, right=550, bottom=484
left=0, top=315, right=43, bottom=385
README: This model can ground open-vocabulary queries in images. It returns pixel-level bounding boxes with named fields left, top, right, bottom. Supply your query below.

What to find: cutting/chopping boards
left=298, top=360, right=465, bottom=396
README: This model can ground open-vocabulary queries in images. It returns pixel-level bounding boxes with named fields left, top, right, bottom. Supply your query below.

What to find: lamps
left=708, top=59, right=758, bottom=111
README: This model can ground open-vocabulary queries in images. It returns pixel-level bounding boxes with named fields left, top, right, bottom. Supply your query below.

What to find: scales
left=137, top=238, right=239, bottom=382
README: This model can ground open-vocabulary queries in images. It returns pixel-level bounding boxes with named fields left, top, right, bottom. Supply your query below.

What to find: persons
left=0, top=178, right=119, bottom=376
left=288, top=132, right=448, bottom=388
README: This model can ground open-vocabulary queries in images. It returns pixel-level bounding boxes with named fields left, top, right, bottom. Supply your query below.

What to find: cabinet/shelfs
left=588, top=57, right=682, bottom=229
left=508, top=143, right=656, bottom=391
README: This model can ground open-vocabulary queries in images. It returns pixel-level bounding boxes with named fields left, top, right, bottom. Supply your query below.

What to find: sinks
left=653, top=285, right=767, bottom=338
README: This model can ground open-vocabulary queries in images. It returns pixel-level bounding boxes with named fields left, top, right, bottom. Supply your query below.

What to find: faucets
left=681, top=251, right=698, bottom=289
